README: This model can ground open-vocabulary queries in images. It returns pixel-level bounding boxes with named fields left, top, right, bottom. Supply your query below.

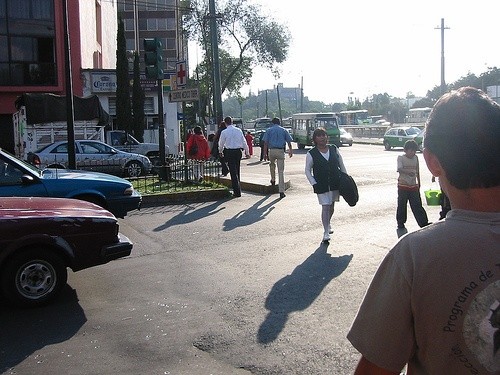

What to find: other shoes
left=280, top=192, right=286, bottom=198
left=323, top=230, right=330, bottom=241
left=270, top=179, right=275, bottom=186
left=397, top=222, right=405, bottom=228
left=329, top=228, right=334, bottom=234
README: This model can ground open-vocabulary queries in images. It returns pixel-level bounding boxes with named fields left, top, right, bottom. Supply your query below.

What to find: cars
left=339, top=128, right=353, bottom=147
left=383, top=126, right=422, bottom=150
left=414, top=128, right=425, bottom=152
left=0, top=147, right=143, bottom=220
left=25, top=139, right=151, bottom=180
left=0, top=196, right=134, bottom=310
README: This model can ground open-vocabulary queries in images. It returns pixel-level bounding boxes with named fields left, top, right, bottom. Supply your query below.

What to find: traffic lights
left=144, top=38, right=163, bottom=79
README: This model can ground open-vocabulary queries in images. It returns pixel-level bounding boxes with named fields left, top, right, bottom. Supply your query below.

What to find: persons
left=186, top=128, right=194, bottom=141
left=245, top=131, right=254, bottom=157
left=347, top=86, right=500, bottom=375
left=185, top=126, right=211, bottom=182
left=261, top=118, right=293, bottom=198
left=396, top=139, right=432, bottom=230
left=304, top=127, right=347, bottom=241
left=217, top=116, right=251, bottom=197
left=210, top=121, right=230, bottom=177
left=259, top=130, right=269, bottom=162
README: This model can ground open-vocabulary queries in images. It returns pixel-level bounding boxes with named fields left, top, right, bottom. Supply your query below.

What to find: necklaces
left=317, top=146, right=327, bottom=149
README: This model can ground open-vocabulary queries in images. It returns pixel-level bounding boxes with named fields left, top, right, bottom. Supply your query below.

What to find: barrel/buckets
left=424, top=181, right=442, bottom=206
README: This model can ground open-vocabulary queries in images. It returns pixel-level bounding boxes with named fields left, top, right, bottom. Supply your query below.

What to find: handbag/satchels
left=190, top=135, right=198, bottom=154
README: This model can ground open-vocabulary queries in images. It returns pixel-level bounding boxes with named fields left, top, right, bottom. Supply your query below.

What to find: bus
left=339, top=110, right=368, bottom=125
left=290, top=112, right=340, bottom=149
left=231, top=117, right=293, bottom=146
left=408, top=106, right=434, bottom=123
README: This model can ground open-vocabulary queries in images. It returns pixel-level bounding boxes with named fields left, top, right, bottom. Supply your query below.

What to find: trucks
left=13, top=104, right=170, bottom=162
